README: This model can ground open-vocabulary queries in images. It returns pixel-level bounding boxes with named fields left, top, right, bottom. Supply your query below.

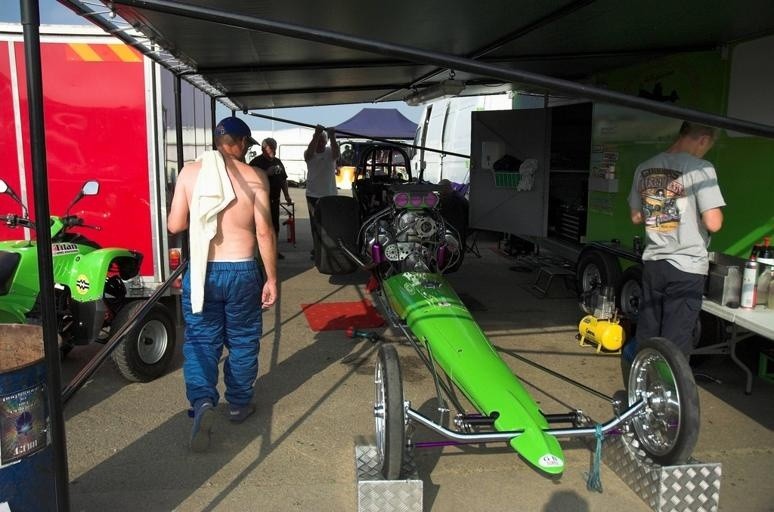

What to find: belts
left=271, top=197, right=281, bottom=203
left=493, top=172, right=521, bottom=189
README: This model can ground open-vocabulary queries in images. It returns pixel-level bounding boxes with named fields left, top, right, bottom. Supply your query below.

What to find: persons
left=249, top=138, right=291, bottom=259
left=304, top=124, right=340, bottom=260
left=168, top=118, right=278, bottom=453
left=627, top=121, right=727, bottom=372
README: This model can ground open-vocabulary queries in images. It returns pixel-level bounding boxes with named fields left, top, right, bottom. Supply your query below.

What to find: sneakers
left=230, top=403, right=256, bottom=424
left=277, top=252, right=285, bottom=259
left=310, top=250, right=316, bottom=262
left=191, top=397, right=215, bottom=452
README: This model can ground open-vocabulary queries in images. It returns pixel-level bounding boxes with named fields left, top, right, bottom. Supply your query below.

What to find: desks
left=691, top=295, right=773, bottom=399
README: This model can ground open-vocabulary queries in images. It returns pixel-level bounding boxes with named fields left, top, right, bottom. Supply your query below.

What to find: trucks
left=409, top=36, right=774, bottom=342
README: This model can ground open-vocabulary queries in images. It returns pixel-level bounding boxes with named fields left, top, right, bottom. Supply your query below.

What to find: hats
left=214, top=117, right=260, bottom=146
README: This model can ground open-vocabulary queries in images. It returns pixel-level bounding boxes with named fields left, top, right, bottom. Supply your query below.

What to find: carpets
left=300, top=298, right=384, bottom=333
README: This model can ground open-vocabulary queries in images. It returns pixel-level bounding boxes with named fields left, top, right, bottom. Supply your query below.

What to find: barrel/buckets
left=0, top=321, right=66, bottom=511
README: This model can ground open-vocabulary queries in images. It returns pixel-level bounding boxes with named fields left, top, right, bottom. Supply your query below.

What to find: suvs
left=0, top=179, right=177, bottom=382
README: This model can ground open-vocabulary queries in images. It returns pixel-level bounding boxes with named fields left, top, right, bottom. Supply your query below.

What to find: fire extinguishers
left=283, top=212, right=295, bottom=242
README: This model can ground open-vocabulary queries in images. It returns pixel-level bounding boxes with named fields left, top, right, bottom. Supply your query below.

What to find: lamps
left=405, top=71, right=467, bottom=108
